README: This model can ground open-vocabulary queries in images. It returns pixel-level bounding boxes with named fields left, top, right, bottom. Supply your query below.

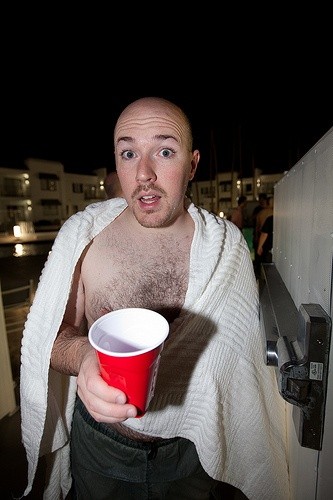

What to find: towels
left=19, top=194, right=293, bottom=500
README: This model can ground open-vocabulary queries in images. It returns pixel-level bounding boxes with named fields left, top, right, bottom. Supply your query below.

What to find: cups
left=88, top=307, right=170, bottom=419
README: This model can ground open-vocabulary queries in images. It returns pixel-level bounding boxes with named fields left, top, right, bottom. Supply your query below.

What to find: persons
left=50, top=97, right=257, bottom=500
left=252, top=192, right=266, bottom=212
left=257, top=215, right=273, bottom=265
left=255, top=197, right=271, bottom=239
left=103, top=170, right=122, bottom=202
left=232, top=195, right=247, bottom=227
